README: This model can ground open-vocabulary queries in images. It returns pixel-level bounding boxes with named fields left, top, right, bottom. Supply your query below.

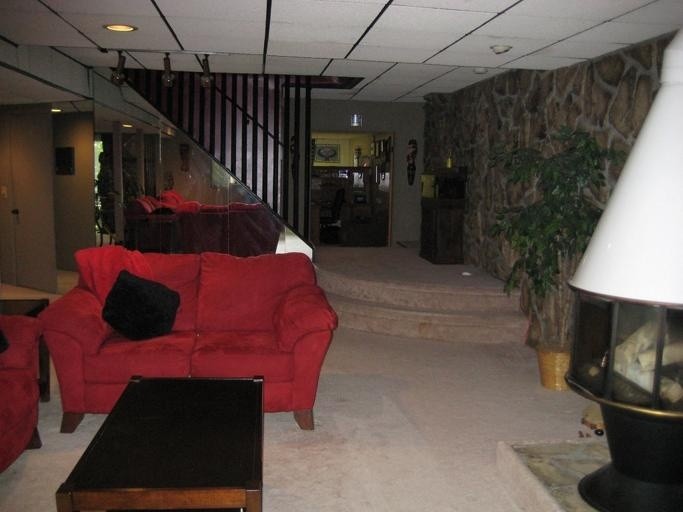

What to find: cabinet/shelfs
left=312, top=166, right=372, bottom=235
left=419, top=197, right=465, bottom=264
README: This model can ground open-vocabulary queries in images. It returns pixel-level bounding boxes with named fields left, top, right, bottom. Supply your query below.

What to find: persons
left=96, top=152, right=117, bottom=235
left=121, top=157, right=144, bottom=204
left=172, top=142, right=202, bottom=190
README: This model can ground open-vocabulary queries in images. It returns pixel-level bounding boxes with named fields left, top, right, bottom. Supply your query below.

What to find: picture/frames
left=371, top=140, right=384, bottom=185
left=54, top=147, right=75, bottom=175
left=314, top=143, right=340, bottom=163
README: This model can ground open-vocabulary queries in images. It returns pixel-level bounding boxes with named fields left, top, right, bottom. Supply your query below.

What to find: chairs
left=39, top=245, right=338, bottom=432
left=320, top=188, right=345, bottom=241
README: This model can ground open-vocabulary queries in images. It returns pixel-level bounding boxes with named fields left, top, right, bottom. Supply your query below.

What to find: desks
left=0, top=299, right=50, bottom=403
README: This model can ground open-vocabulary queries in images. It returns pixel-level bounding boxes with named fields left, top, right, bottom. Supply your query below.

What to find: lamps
left=110, top=49, right=215, bottom=89
left=565, top=25, right=683, bottom=512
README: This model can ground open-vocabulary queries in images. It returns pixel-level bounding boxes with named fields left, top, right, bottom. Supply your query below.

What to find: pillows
left=200, top=204, right=228, bottom=213
left=198, top=252, right=316, bottom=334
left=228, top=202, right=263, bottom=210
left=75, top=246, right=200, bottom=333
left=102, top=270, right=180, bottom=341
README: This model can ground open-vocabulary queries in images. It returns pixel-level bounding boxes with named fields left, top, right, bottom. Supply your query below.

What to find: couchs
left=0, top=316, right=42, bottom=474
left=132, top=190, right=281, bottom=256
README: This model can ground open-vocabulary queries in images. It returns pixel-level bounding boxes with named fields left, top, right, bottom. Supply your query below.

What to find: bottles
left=446, top=151, right=452, bottom=169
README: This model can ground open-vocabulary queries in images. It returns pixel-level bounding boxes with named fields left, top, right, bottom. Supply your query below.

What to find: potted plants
left=489, top=123, right=629, bottom=395
